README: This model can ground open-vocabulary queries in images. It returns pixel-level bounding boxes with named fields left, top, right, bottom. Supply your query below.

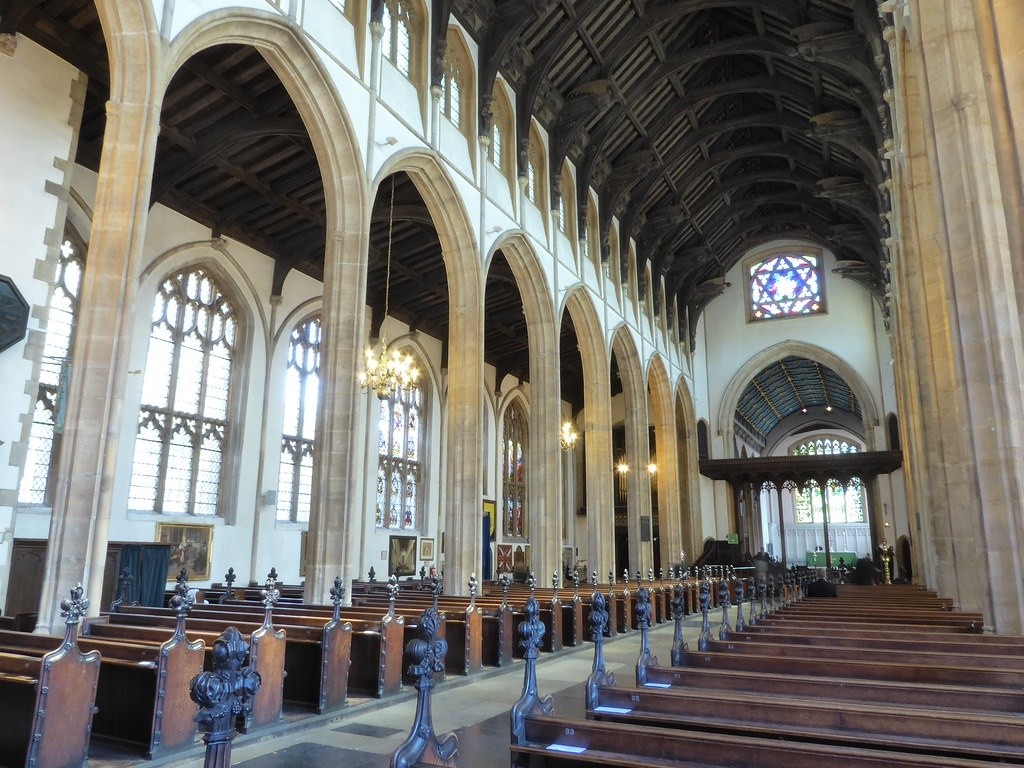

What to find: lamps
left=354, top=171, right=430, bottom=403
left=557, top=418, right=582, bottom=453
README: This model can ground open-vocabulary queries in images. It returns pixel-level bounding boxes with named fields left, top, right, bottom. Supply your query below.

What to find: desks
left=806, top=550, right=858, bottom=567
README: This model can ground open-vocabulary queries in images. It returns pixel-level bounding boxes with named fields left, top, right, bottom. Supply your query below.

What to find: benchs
left=0, top=564, right=1024, bottom=768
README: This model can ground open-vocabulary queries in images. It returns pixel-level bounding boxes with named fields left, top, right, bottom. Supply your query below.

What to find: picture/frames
left=387, top=534, right=418, bottom=577
left=155, top=521, right=214, bottom=582
left=420, top=538, right=435, bottom=561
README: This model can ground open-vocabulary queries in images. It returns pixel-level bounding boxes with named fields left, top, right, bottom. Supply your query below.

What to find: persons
left=753, top=547, right=770, bottom=562
left=807, top=568, right=839, bottom=598
